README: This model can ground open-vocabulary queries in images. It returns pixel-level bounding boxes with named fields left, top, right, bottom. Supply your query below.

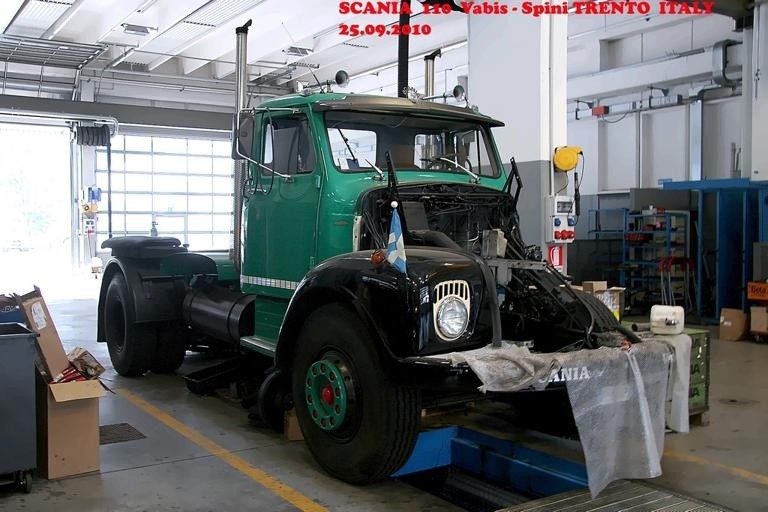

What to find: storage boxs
left=750, top=307, right=767, bottom=335
left=9, top=285, right=71, bottom=379
left=36, top=377, right=116, bottom=480
left=582, top=281, right=625, bottom=323
left=719, top=308, right=747, bottom=341
left=66, top=347, right=105, bottom=381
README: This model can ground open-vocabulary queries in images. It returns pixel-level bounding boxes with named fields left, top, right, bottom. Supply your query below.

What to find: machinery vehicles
left=98, top=17, right=676, bottom=485
